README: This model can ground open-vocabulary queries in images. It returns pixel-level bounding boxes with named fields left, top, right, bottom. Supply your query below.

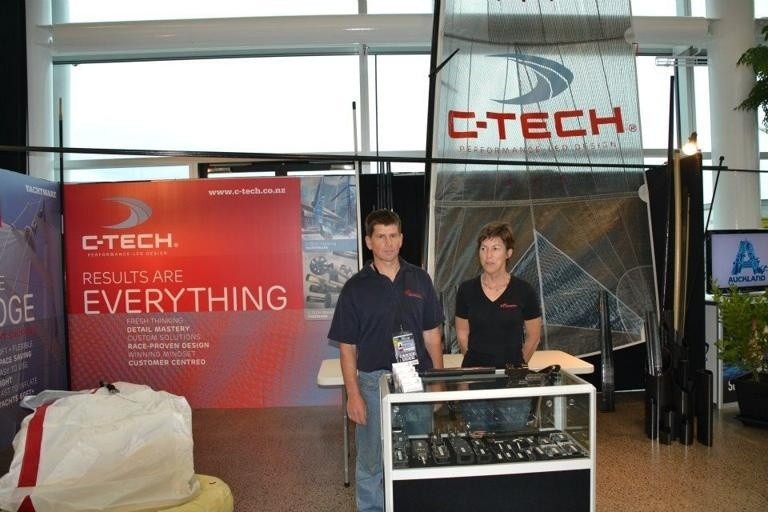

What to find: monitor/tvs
left=704, top=228, right=768, bottom=294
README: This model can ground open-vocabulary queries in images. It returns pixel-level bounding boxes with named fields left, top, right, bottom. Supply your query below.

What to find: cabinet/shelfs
left=378, top=373, right=597, bottom=512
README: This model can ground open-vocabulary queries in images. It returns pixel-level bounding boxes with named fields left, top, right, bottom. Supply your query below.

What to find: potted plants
left=708, top=272, right=768, bottom=430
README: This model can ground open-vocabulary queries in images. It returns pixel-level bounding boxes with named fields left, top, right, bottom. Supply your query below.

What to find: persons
left=454, top=220, right=542, bottom=432
left=328, top=209, right=446, bottom=510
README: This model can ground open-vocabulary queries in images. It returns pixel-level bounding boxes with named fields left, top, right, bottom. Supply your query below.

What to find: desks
left=316, top=348, right=597, bottom=488
